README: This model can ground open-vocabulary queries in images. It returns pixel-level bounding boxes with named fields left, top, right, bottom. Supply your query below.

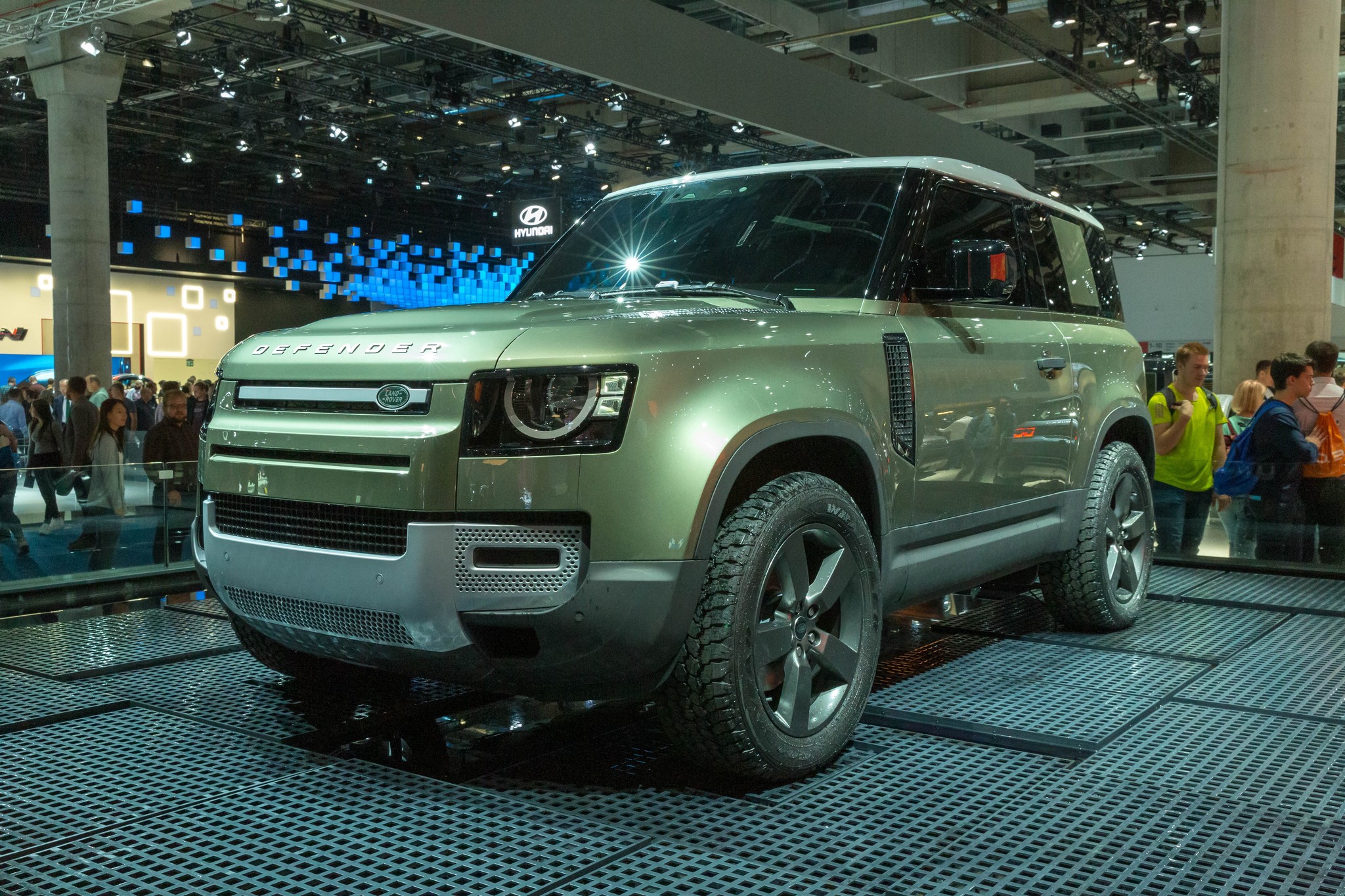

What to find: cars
left=114, top=373, right=158, bottom=394
left=14, top=368, right=54, bottom=388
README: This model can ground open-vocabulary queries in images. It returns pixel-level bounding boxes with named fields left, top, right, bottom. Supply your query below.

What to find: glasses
left=167, top=403, right=187, bottom=409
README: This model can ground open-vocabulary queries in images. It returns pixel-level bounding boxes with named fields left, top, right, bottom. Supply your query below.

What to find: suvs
left=190, top=155, right=1159, bottom=791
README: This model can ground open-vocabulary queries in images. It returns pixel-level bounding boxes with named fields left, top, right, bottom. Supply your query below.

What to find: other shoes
left=67, top=531, right=98, bottom=551
left=50, top=517, right=65, bottom=530
left=39, top=523, right=51, bottom=535
left=89, top=555, right=116, bottom=571
left=19, top=540, right=30, bottom=555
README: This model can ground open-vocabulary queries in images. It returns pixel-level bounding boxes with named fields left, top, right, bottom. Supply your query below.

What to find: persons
left=87, top=398, right=129, bottom=572
left=65, top=376, right=101, bottom=550
left=1289, top=339, right=1345, bottom=568
left=1250, top=349, right=1329, bottom=563
left=84, top=374, right=220, bottom=472
left=0, top=374, right=75, bottom=558
left=1145, top=341, right=1231, bottom=558
left=143, top=390, right=199, bottom=565
left=1219, top=359, right=1277, bottom=558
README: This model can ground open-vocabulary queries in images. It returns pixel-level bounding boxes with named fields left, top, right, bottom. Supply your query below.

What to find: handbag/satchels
left=56, top=473, right=76, bottom=496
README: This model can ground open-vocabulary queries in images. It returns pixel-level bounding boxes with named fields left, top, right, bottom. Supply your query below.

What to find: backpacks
left=1223, top=417, right=1239, bottom=449
left=1299, top=387, right=1345, bottom=478
left=1214, top=400, right=1289, bottom=497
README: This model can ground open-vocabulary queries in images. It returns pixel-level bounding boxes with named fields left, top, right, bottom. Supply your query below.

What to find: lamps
left=0, top=0, right=1218, bottom=260
